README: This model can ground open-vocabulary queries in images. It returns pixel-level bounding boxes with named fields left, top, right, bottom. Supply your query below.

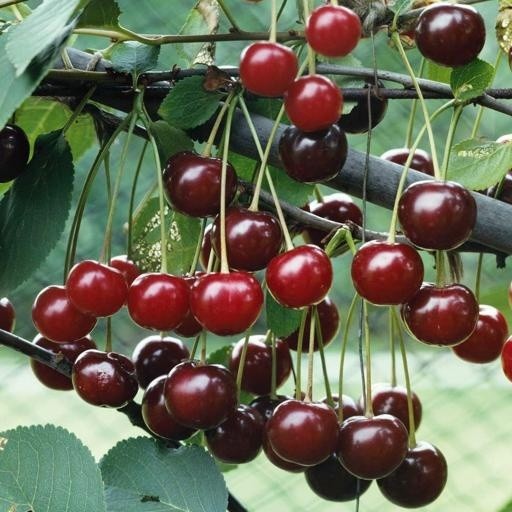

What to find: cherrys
left=0, top=0, right=512, bottom=509
